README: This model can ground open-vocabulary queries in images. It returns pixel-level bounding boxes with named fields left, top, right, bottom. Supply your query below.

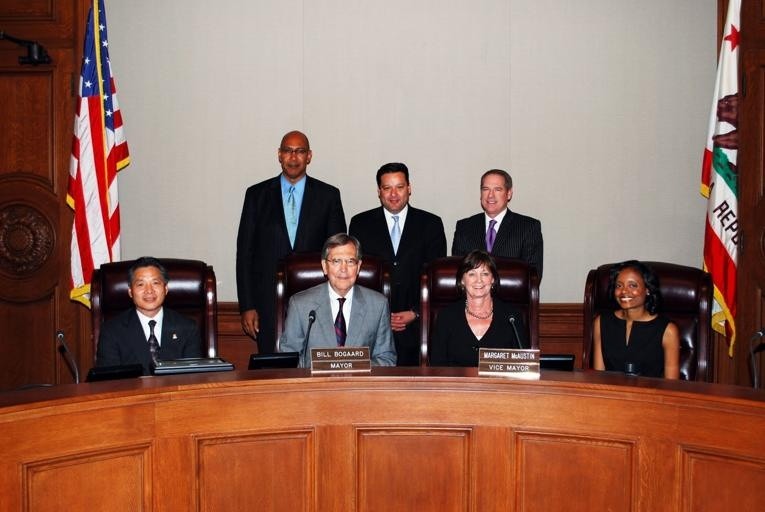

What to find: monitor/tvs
left=248, top=352, right=299, bottom=370
left=540, top=353, right=575, bottom=372
left=83, top=363, right=144, bottom=382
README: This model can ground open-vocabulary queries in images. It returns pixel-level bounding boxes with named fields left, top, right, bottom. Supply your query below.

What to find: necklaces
left=466, top=300, right=493, bottom=319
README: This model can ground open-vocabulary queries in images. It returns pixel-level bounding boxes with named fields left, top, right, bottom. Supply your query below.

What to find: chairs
left=579, top=261, right=713, bottom=382
left=88, top=257, right=220, bottom=362
left=275, top=258, right=395, bottom=354
left=417, top=255, right=540, bottom=368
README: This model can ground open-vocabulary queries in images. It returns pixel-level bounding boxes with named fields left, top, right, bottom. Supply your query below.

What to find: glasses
left=326, top=257, right=359, bottom=267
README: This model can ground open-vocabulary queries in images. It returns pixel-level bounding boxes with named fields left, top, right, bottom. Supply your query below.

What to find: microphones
left=748, top=328, right=765, bottom=388
left=504, top=312, right=523, bottom=350
left=301, top=310, right=316, bottom=368
left=55, top=328, right=79, bottom=383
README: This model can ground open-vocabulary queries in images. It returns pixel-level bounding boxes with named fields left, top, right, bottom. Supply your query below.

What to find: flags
left=701, top=1, right=742, bottom=357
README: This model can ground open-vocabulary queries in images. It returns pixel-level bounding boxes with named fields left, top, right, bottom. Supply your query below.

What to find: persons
left=97, top=258, right=202, bottom=376
left=428, top=249, right=524, bottom=366
left=452, top=170, right=543, bottom=286
left=593, top=261, right=680, bottom=380
left=66, top=1, right=130, bottom=310
left=236, top=132, right=347, bottom=355
left=348, top=162, right=447, bottom=366
left=280, top=233, right=397, bottom=368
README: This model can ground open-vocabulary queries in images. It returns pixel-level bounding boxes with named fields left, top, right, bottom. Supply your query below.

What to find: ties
left=334, top=297, right=347, bottom=346
left=392, top=215, right=400, bottom=254
left=286, top=186, right=296, bottom=242
left=148, top=319, right=161, bottom=364
left=484, top=219, right=497, bottom=254
